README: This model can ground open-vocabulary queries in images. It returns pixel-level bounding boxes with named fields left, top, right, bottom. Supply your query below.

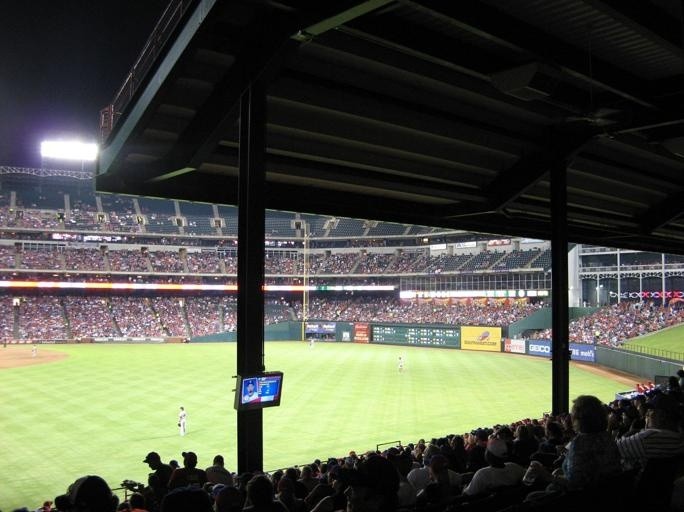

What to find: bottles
left=522, top=461, right=542, bottom=486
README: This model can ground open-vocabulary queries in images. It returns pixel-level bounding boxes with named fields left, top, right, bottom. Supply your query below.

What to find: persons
left=397, top=356, right=404, bottom=374
left=1, top=370, right=684, bottom=512
left=2, top=185, right=684, bottom=346
left=30, top=343, right=37, bottom=357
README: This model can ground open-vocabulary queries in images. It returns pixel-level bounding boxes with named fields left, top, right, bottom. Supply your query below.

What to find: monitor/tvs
left=234, top=371, right=284, bottom=410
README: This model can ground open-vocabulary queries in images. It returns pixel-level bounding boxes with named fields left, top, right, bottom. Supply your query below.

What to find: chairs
left=222, top=295, right=288, bottom=336
left=325, top=247, right=553, bottom=274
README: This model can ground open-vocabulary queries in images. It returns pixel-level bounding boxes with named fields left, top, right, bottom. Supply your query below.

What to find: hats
left=143, top=452, right=159, bottom=463
left=486, top=438, right=509, bottom=459
left=170, top=460, right=179, bottom=467
left=641, top=395, right=679, bottom=410
left=182, top=451, right=197, bottom=463
left=55, top=474, right=119, bottom=511
left=471, top=430, right=488, bottom=441
left=336, top=456, right=401, bottom=493
left=247, top=380, right=254, bottom=387
left=44, top=502, right=53, bottom=506
